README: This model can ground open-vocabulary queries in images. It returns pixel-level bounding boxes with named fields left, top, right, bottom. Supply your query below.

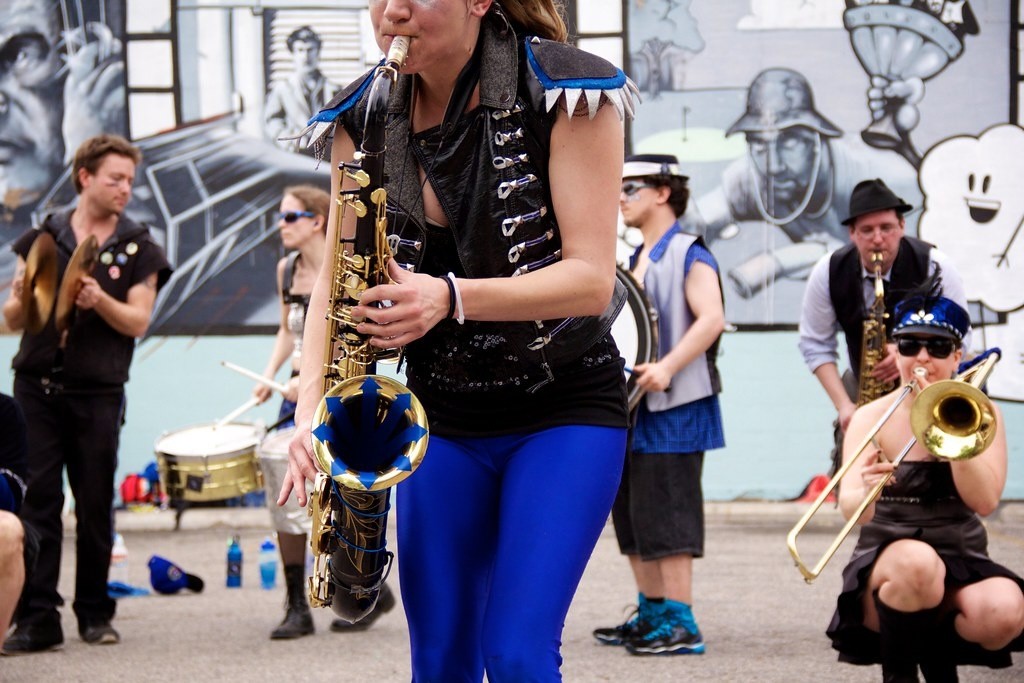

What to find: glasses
left=622, top=182, right=656, bottom=195
left=280, top=211, right=314, bottom=222
left=894, top=335, right=955, bottom=358
left=857, top=221, right=900, bottom=237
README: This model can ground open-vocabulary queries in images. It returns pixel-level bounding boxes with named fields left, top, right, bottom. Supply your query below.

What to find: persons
left=256, top=185, right=396, bottom=639
left=797, top=178, right=972, bottom=511
left=0, top=509, right=25, bottom=656
left=824, top=292, right=1024, bottom=683
left=2, top=133, right=173, bottom=654
left=276, top=1, right=644, bottom=683
left=593, top=155, right=728, bottom=656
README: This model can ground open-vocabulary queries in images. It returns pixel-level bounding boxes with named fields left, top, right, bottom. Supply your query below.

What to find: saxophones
left=307, top=35, right=431, bottom=623
left=853, top=248, right=895, bottom=405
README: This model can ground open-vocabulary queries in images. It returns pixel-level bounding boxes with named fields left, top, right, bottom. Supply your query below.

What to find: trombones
left=787, top=352, right=999, bottom=583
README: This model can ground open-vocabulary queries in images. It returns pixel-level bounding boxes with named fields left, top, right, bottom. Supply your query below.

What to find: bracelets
left=440, top=271, right=464, bottom=324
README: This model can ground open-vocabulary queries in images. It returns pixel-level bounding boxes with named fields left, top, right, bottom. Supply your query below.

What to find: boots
left=872, top=587, right=1013, bottom=683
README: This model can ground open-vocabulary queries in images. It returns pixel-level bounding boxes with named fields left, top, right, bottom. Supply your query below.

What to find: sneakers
left=593, top=604, right=657, bottom=644
left=625, top=618, right=703, bottom=656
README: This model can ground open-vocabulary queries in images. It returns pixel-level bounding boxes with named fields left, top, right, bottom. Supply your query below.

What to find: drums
left=610, top=263, right=659, bottom=414
left=153, top=421, right=260, bottom=503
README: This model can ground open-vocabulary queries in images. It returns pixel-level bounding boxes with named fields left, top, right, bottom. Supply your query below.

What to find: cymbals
left=55, top=234, right=99, bottom=336
left=21, top=233, right=58, bottom=337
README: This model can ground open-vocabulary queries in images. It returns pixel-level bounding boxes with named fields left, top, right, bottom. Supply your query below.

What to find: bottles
left=111, top=535, right=127, bottom=584
left=227, top=535, right=242, bottom=587
left=305, top=547, right=314, bottom=587
left=260, top=535, right=276, bottom=589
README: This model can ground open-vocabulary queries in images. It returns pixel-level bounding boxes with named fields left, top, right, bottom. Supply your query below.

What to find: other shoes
left=333, top=582, right=395, bottom=631
left=80, top=624, right=117, bottom=643
left=2, top=627, right=65, bottom=657
left=271, top=604, right=314, bottom=640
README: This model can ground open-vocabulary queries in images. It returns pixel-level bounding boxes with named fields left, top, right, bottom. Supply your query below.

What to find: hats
left=148, top=555, right=205, bottom=594
left=623, top=153, right=689, bottom=180
left=841, top=179, right=912, bottom=226
left=891, top=296, right=971, bottom=343
